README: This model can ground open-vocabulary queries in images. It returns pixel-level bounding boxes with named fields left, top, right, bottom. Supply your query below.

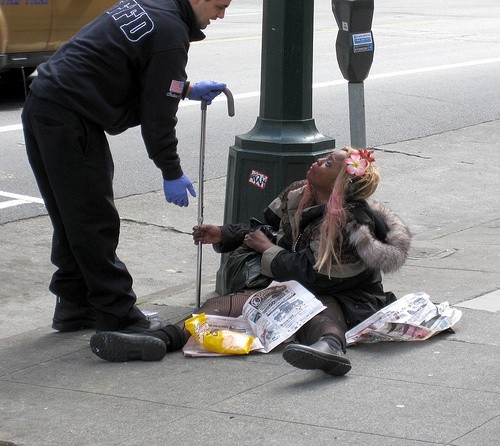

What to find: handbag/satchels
left=220, top=216, right=283, bottom=291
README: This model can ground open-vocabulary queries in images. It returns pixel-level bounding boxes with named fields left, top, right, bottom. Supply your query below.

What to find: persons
left=90, top=147, right=412, bottom=376
left=22, top=1, right=233, bottom=334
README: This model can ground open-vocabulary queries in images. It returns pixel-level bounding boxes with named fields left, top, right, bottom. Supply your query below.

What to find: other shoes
left=89, top=302, right=162, bottom=333
left=52, top=298, right=97, bottom=331
left=282, top=336, right=351, bottom=376
left=88, top=327, right=167, bottom=361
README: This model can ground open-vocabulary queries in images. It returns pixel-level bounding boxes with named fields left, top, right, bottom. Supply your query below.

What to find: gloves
left=163, top=174, right=196, bottom=207
left=188, top=81, right=226, bottom=104
